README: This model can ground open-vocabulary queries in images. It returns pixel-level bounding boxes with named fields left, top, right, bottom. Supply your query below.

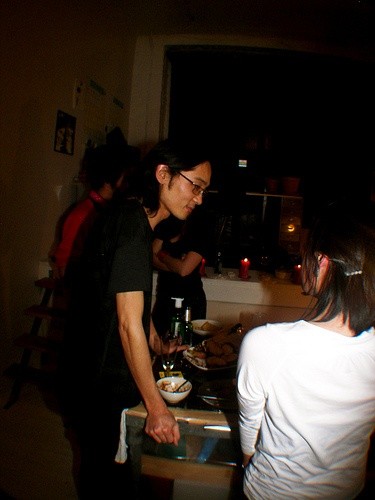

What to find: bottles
left=170, top=299, right=184, bottom=338
left=215, top=252, right=222, bottom=274
left=177, top=307, right=193, bottom=361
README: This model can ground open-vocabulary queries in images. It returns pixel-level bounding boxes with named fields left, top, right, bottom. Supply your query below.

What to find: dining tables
left=125, top=378, right=247, bottom=484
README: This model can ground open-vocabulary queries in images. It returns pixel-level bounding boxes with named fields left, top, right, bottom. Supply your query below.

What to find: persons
left=53, top=135, right=214, bottom=500
left=236, top=214, right=375, bottom=500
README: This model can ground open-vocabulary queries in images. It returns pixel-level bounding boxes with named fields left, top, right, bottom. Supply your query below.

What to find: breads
left=205, top=332, right=237, bottom=365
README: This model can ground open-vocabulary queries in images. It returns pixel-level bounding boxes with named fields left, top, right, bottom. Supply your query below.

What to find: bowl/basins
left=157, top=377, right=192, bottom=403
left=190, top=319, right=223, bottom=335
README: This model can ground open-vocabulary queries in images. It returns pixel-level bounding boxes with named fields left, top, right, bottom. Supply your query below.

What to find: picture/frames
left=54, top=110, right=77, bottom=156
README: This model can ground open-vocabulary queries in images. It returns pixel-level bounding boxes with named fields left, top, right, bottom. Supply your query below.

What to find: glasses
left=170, top=167, right=210, bottom=196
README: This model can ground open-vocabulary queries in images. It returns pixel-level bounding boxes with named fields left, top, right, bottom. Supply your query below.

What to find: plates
left=182, top=346, right=238, bottom=371
